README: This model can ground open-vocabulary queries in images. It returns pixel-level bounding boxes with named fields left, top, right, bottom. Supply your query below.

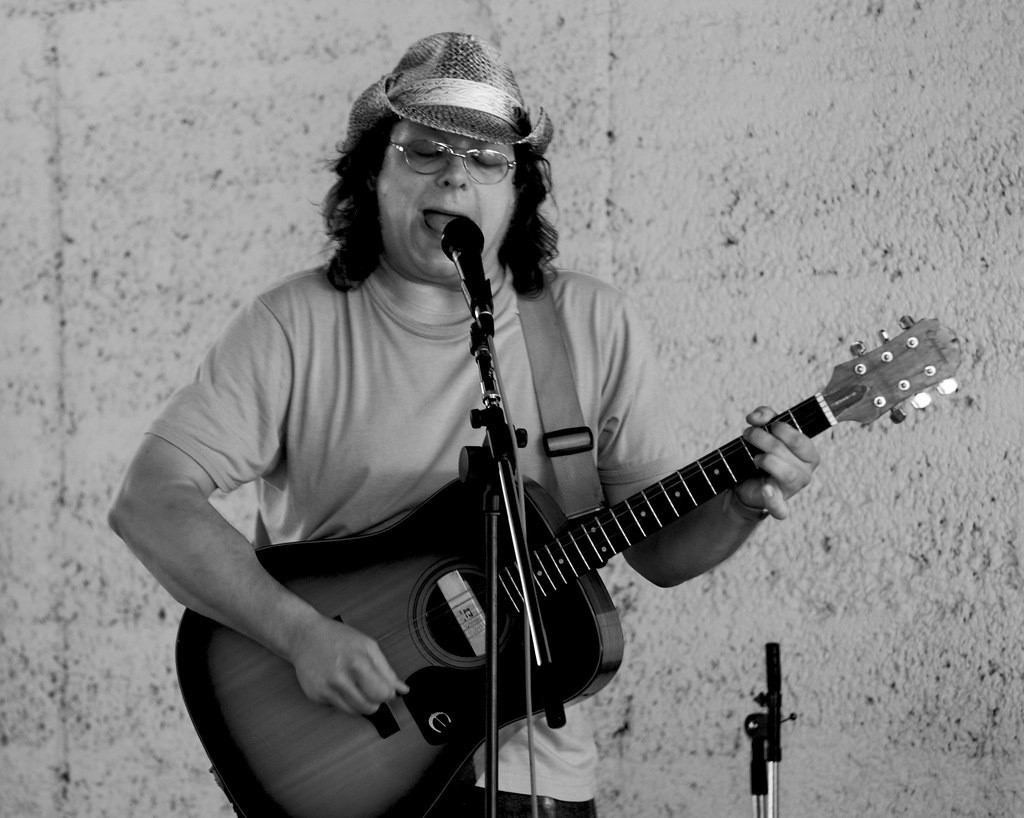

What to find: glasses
left=388, top=139, right=517, bottom=185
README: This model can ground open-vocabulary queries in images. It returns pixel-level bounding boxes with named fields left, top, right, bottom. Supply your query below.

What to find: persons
left=104, top=32, right=821, bottom=818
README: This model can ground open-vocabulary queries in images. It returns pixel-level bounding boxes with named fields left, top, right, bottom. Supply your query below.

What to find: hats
left=335, top=32, right=553, bottom=154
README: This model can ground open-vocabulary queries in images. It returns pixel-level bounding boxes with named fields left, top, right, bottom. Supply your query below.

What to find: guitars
left=174, top=313, right=963, bottom=818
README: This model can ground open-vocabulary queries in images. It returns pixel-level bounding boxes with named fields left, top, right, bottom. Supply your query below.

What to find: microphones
left=441, top=217, right=494, bottom=342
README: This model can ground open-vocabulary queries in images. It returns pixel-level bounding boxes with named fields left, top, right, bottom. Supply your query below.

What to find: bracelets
left=733, top=490, right=769, bottom=521
